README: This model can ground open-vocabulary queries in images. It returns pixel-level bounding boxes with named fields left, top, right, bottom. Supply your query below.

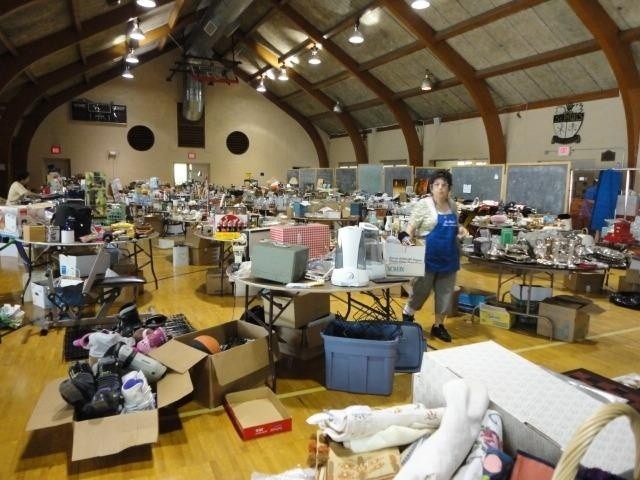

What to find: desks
left=254, top=182, right=561, bottom=233
left=0, top=183, right=253, bottom=309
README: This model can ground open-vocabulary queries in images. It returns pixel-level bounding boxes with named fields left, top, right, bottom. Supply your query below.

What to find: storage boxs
left=1, top=221, right=640, bottom=477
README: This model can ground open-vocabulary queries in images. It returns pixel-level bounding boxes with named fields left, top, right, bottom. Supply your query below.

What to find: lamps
left=349, top=13, right=365, bottom=44
left=421, top=74, right=433, bottom=92
left=308, top=44, right=322, bottom=65
left=125, top=47, right=139, bottom=63
left=120, top=65, right=135, bottom=79
left=130, top=20, right=145, bottom=40
left=333, top=98, right=343, bottom=113
left=278, top=62, right=290, bottom=81
left=256, top=77, right=268, bottom=93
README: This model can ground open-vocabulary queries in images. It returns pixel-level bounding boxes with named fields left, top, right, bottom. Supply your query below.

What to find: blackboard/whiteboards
left=415, top=167, right=446, bottom=193
left=384, top=165, right=413, bottom=198
left=299, top=168, right=317, bottom=189
left=504, top=160, right=572, bottom=219
left=449, top=164, right=505, bottom=201
left=335, top=168, right=357, bottom=196
left=286, top=169, right=299, bottom=187
left=317, top=168, right=334, bottom=189
left=357, top=163, right=384, bottom=195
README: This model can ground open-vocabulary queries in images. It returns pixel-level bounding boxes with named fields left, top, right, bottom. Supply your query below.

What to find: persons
left=5, top=170, right=42, bottom=206
left=402, top=169, right=459, bottom=342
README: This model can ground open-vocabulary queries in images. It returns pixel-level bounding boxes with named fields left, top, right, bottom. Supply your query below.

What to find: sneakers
left=430, top=324, right=451, bottom=342
left=402, top=312, right=414, bottom=322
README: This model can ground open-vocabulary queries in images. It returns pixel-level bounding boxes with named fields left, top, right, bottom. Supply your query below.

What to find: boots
left=59, top=302, right=167, bottom=421
